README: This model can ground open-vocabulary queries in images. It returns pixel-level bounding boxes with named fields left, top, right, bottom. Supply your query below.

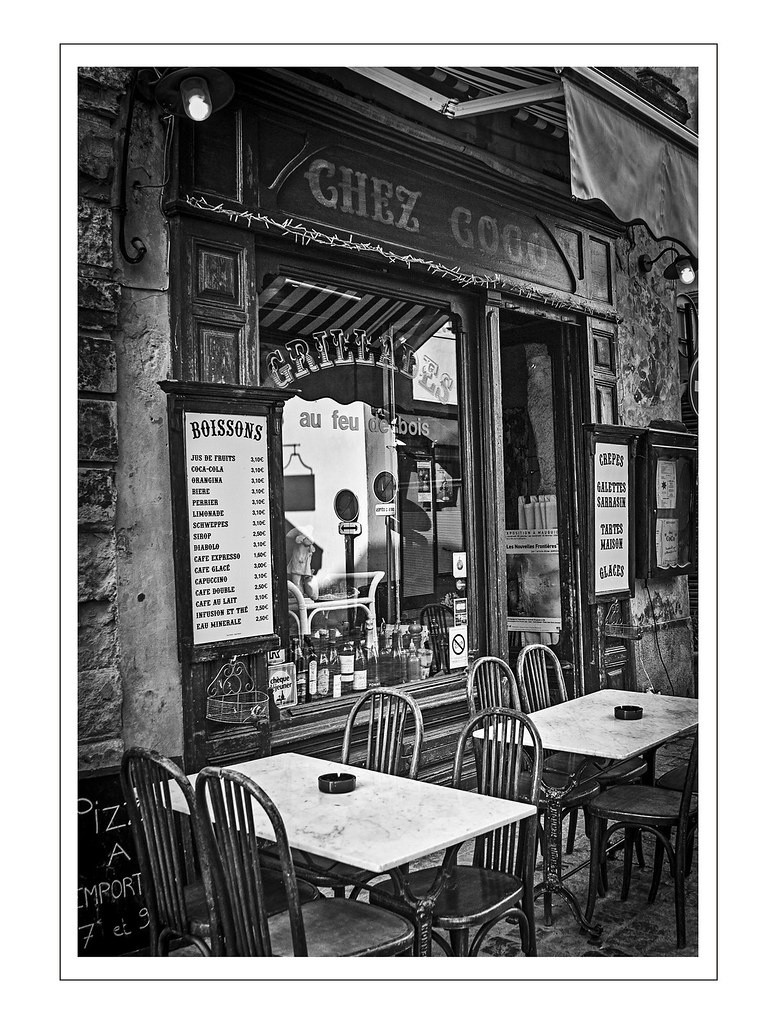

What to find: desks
left=473, top=688, right=698, bottom=926
left=133, top=752, right=537, bottom=957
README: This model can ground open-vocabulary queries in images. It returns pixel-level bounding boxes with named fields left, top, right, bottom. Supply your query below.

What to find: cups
left=418, top=649, right=433, bottom=680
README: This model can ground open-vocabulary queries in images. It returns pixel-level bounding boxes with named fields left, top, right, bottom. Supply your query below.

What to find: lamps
left=137, top=68, right=235, bottom=122
left=638, top=247, right=698, bottom=285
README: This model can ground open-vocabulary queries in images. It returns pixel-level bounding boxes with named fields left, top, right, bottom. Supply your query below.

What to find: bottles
left=327, top=631, right=341, bottom=697
left=365, top=620, right=380, bottom=689
left=304, top=636, right=317, bottom=700
left=292, top=639, right=306, bottom=703
left=376, top=620, right=393, bottom=686
left=391, top=620, right=407, bottom=685
left=341, top=623, right=353, bottom=692
left=319, top=632, right=328, bottom=697
left=351, top=627, right=367, bottom=692
left=407, top=640, right=419, bottom=684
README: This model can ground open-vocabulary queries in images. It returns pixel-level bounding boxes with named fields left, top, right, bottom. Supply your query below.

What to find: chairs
left=121, top=643, right=698, bottom=957
left=288, top=571, right=385, bottom=659
